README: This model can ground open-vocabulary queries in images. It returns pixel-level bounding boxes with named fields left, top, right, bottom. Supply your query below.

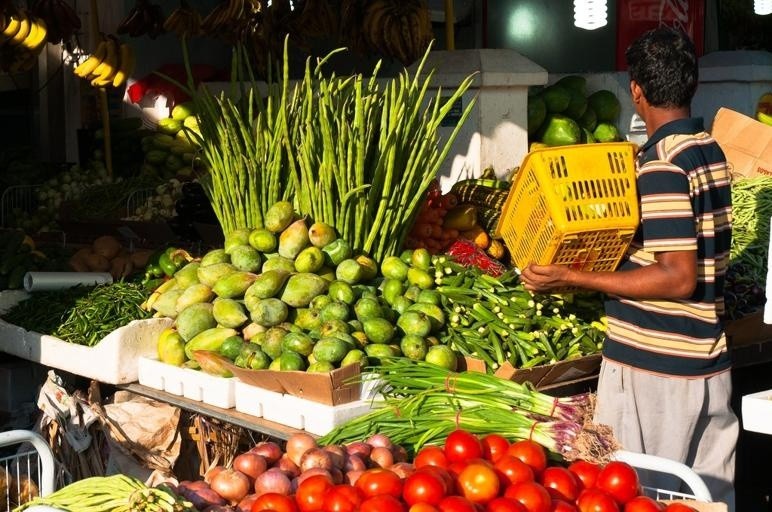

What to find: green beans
left=10, top=473, right=199, bottom=512
left=729, top=175, right=772, bottom=290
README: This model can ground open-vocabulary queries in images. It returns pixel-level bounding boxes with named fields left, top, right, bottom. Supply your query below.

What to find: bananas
left=0, top=1, right=80, bottom=74
left=202, top=0, right=263, bottom=42
left=166, top=2, right=202, bottom=38
left=74, top=35, right=137, bottom=88
left=361, top=1, right=436, bottom=68
left=118, top=2, right=163, bottom=36
left=287, top=0, right=358, bottom=41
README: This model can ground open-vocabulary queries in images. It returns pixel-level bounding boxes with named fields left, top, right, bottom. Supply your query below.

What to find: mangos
left=143, top=201, right=460, bottom=376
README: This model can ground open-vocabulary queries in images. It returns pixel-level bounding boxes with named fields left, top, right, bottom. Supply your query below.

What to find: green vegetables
left=148, top=28, right=481, bottom=268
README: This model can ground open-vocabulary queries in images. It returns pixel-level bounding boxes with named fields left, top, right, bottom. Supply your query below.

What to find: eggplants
left=725, top=260, right=765, bottom=320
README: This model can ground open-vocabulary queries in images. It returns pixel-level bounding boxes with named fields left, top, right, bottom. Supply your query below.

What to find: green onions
left=316, top=355, right=619, bottom=466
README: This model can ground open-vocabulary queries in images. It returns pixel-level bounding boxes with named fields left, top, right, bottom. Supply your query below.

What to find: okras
left=430, top=253, right=606, bottom=376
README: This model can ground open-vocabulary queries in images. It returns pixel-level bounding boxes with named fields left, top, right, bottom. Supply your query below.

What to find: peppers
left=0, top=276, right=156, bottom=346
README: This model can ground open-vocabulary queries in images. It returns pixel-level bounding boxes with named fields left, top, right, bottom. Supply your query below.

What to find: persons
left=520, top=29, right=732, bottom=512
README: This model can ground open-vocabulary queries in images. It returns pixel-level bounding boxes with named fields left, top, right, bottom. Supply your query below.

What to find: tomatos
left=251, top=427, right=662, bottom=511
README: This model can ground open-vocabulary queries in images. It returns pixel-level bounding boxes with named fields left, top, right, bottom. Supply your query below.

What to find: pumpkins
left=443, top=181, right=510, bottom=261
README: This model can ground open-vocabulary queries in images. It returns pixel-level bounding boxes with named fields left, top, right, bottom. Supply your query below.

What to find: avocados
left=527, top=76, right=621, bottom=147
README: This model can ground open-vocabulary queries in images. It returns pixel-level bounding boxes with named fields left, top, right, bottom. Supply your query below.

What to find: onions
left=154, top=431, right=414, bottom=512
